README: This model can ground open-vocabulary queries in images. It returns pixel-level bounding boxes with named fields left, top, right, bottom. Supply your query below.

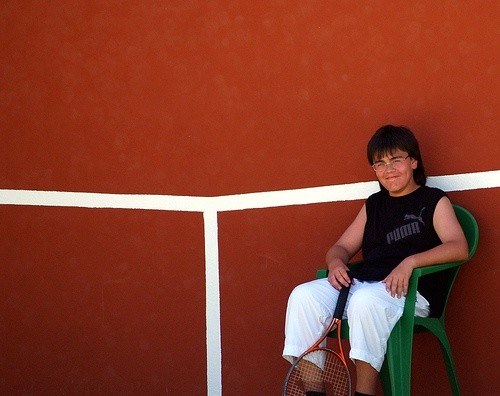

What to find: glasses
left=371, top=155, right=410, bottom=171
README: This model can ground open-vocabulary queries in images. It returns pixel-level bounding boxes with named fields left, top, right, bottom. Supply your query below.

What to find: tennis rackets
left=280, top=269, right=353, bottom=396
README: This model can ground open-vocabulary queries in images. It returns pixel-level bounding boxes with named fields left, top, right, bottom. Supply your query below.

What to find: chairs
left=316, top=203, right=479, bottom=396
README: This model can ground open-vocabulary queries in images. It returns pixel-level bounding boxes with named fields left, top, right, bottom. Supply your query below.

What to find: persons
left=282, top=125, right=470, bottom=396
